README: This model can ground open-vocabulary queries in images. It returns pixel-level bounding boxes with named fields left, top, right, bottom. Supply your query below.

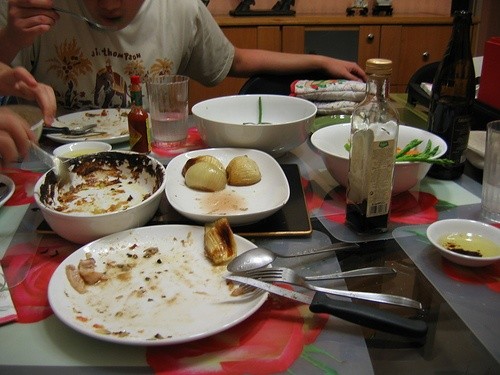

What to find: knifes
left=40, top=129, right=108, bottom=135
left=222, top=276, right=430, bottom=340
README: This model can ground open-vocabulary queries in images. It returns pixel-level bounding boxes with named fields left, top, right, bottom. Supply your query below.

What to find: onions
left=182, top=154, right=261, bottom=192
left=204, top=216, right=237, bottom=264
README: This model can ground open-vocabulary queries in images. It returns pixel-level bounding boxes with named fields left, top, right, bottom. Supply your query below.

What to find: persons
left=0, top=0, right=369, bottom=112
left=0, top=62, right=57, bottom=164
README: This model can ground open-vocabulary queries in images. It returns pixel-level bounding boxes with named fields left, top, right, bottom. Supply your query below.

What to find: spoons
left=17, top=5, right=118, bottom=33
left=28, top=140, right=71, bottom=188
left=42, top=123, right=97, bottom=135
left=226, top=241, right=360, bottom=273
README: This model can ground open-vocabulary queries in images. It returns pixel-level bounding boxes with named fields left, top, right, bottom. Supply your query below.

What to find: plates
left=0, top=173, right=16, bottom=209
left=44, top=108, right=150, bottom=144
left=48, top=225, right=274, bottom=347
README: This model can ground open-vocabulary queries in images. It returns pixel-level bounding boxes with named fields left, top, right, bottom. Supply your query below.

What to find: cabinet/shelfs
left=187, top=24, right=480, bottom=115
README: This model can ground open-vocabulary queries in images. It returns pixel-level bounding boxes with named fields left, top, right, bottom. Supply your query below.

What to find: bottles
left=127, top=75, right=152, bottom=156
left=345, top=58, right=399, bottom=237
left=425, top=10, right=476, bottom=182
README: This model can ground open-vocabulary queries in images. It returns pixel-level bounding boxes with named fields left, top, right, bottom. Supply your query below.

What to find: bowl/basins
left=3, top=105, right=45, bottom=143
left=33, top=148, right=291, bottom=245
left=53, top=141, right=112, bottom=162
left=191, top=94, right=447, bottom=197
left=426, top=219, right=500, bottom=267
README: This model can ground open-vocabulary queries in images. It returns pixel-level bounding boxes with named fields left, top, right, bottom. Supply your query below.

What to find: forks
left=237, top=264, right=425, bottom=313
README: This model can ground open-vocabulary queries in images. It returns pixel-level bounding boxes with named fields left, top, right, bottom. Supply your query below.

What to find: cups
left=480, top=120, right=500, bottom=225
left=147, top=74, right=190, bottom=142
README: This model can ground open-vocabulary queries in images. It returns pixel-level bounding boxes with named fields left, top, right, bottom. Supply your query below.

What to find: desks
left=0, top=92, right=500, bottom=375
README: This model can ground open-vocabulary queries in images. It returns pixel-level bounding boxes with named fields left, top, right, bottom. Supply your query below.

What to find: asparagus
left=344, top=138, right=455, bottom=165
